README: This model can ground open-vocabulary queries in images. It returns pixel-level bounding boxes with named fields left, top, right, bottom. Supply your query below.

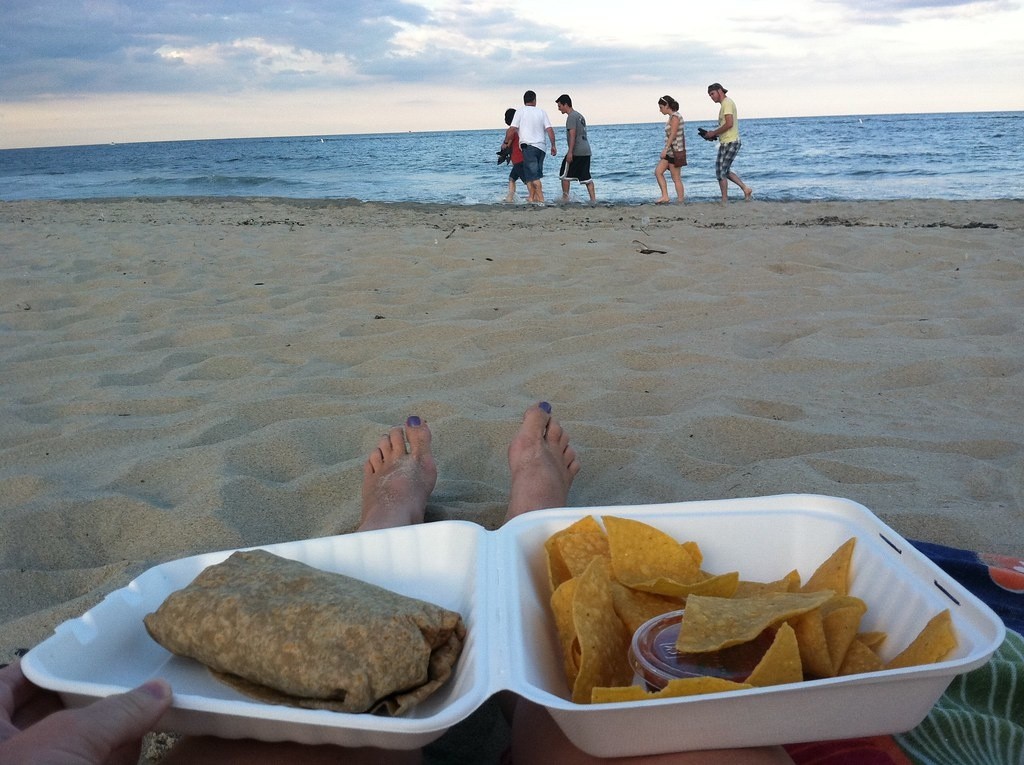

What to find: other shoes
left=697, top=128, right=717, bottom=142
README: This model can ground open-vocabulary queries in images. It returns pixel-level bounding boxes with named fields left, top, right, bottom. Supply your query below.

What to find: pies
left=143, top=549, right=465, bottom=716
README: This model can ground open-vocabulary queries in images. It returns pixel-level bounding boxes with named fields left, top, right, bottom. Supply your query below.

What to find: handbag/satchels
left=673, top=150, right=687, bottom=167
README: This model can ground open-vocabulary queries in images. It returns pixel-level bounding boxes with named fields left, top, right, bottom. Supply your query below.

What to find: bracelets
left=504, top=141, right=508, bottom=144
left=665, top=145, right=669, bottom=148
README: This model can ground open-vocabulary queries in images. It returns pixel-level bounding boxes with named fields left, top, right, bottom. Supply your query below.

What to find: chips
left=544, top=514, right=956, bottom=705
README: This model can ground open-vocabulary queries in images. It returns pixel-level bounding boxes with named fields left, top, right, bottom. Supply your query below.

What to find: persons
left=655, top=96, right=687, bottom=203
left=500, top=91, right=557, bottom=202
left=555, top=95, right=595, bottom=201
left=0, top=401, right=798, bottom=764
left=705, top=83, right=753, bottom=200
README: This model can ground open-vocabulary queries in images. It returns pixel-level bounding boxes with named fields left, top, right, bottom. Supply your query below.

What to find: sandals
left=496, top=146, right=512, bottom=165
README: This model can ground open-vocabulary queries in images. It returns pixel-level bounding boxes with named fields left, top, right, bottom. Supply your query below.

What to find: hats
left=708, top=83, right=727, bottom=94
